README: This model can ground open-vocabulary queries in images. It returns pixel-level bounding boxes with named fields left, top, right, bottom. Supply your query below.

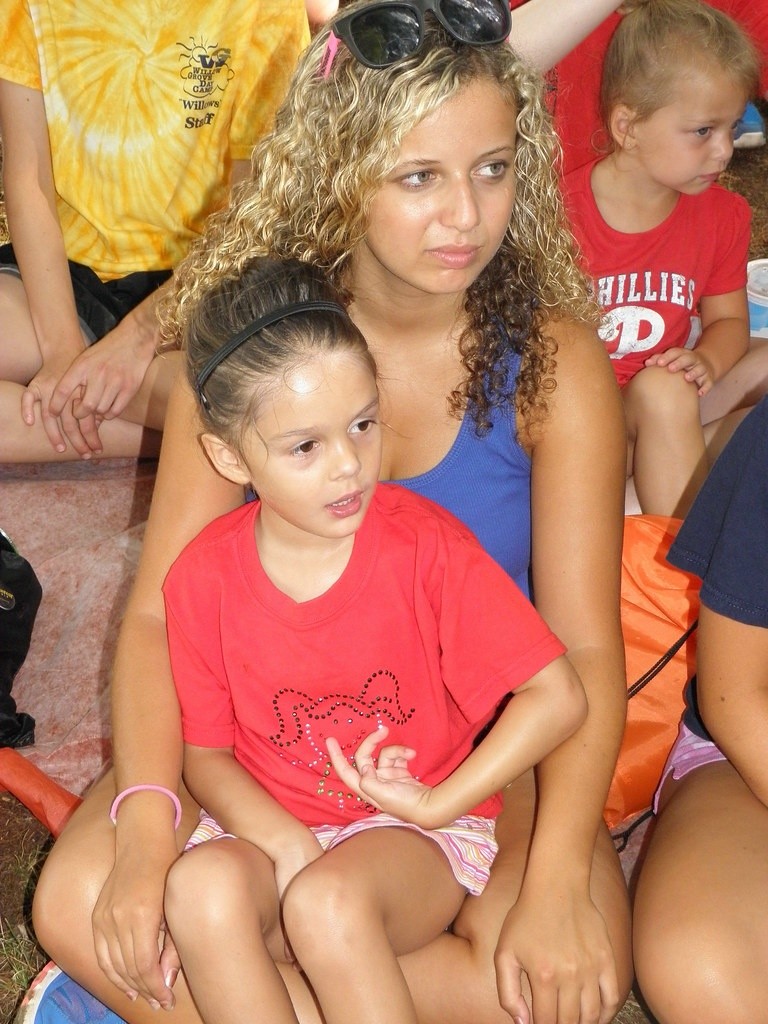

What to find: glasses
left=320, top=0, right=512, bottom=80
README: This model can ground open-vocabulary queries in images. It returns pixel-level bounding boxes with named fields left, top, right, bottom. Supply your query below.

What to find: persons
left=0, top=1, right=310, bottom=465
left=161, top=254, right=591, bottom=1024
left=631, top=394, right=767, bottom=1024
left=506, top=1, right=768, bottom=518
left=14, top=0, right=634, bottom=1024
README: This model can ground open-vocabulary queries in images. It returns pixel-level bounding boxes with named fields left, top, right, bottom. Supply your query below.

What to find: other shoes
left=733, top=101, right=766, bottom=149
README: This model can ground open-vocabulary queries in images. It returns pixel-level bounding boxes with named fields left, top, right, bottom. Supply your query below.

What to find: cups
left=745, top=258, right=768, bottom=330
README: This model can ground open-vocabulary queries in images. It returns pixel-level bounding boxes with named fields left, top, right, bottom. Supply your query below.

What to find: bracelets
left=109, top=783, right=182, bottom=831
